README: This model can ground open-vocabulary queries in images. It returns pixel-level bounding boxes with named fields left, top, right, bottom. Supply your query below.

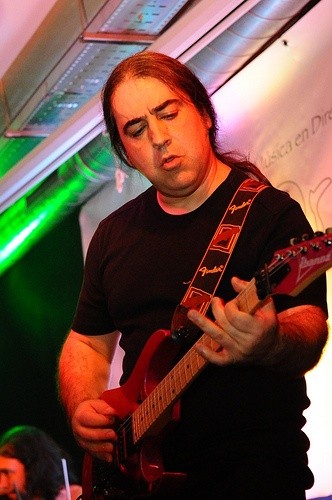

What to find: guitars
left=81, top=228, right=332, bottom=500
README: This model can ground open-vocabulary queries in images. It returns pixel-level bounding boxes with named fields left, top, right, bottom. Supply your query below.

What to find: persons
left=58, top=49, right=329, bottom=499
left=0, top=425, right=82, bottom=500
